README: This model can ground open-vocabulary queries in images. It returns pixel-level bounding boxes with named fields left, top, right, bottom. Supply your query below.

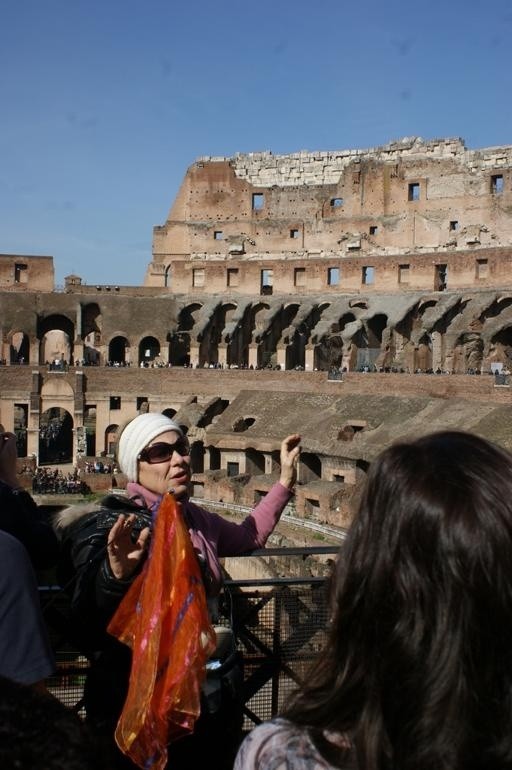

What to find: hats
left=119, top=412, right=184, bottom=483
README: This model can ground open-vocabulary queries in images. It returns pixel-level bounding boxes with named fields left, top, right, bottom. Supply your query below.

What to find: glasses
left=137, top=435, right=190, bottom=465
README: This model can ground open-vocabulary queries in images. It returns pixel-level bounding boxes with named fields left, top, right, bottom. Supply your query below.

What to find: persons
left=0, top=423, right=68, bottom=769
left=45, top=360, right=67, bottom=370
left=53, top=413, right=301, bottom=770
left=314, top=363, right=410, bottom=374
left=76, top=360, right=170, bottom=368
left=19, top=357, right=25, bottom=364
left=184, top=360, right=281, bottom=370
left=292, top=364, right=304, bottom=371
left=232, top=428, right=512, bottom=770
left=415, top=366, right=510, bottom=376
left=31, top=457, right=119, bottom=496
